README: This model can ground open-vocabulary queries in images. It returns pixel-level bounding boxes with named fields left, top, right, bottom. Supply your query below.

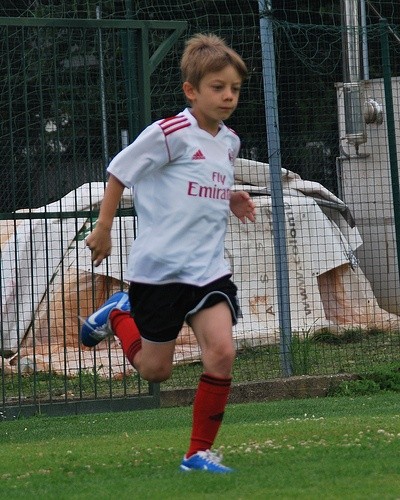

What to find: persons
left=81, top=37, right=260, bottom=479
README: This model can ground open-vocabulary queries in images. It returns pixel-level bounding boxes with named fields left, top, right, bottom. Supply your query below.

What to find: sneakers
left=179, top=451, right=235, bottom=475
left=80, top=292, right=132, bottom=346
